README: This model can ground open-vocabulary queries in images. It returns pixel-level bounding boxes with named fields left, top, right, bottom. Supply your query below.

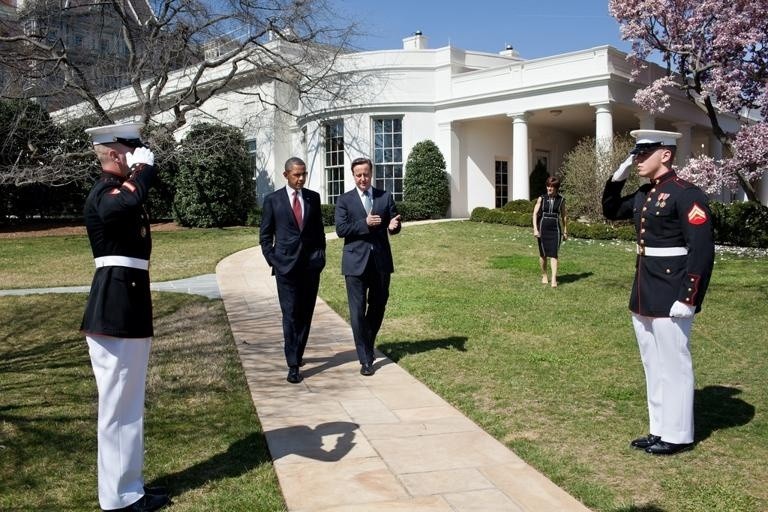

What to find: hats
left=83, top=121, right=149, bottom=150
left=629, top=128, right=683, bottom=154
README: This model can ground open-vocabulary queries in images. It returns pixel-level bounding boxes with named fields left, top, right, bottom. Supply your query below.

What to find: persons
left=81, top=118, right=168, bottom=511
left=531, top=177, right=567, bottom=288
left=258, top=156, right=325, bottom=382
left=333, top=157, right=402, bottom=377
left=601, top=128, right=714, bottom=456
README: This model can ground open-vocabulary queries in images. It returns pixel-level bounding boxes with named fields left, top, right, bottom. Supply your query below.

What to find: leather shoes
left=143, top=485, right=171, bottom=497
left=298, top=356, right=305, bottom=367
left=645, top=438, right=696, bottom=456
left=369, top=351, right=376, bottom=363
left=286, top=366, right=301, bottom=383
left=102, top=494, right=170, bottom=512
left=360, top=363, right=375, bottom=375
left=629, top=434, right=661, bottom=450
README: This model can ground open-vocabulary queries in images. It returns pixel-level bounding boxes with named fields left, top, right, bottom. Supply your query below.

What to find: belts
left=635, top=242, right=690, bottom=258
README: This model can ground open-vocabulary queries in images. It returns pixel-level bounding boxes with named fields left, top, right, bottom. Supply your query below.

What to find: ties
left=292, top=191, right=303, bottom=232
left=362, top=190, right=373, bottom=217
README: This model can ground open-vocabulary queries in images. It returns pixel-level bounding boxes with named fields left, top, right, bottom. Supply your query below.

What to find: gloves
left=668, top=299, right=698, bottom=320
left=125, top=146, right=155, bottom=169
left=610, top=154, right=636, bottom=183
